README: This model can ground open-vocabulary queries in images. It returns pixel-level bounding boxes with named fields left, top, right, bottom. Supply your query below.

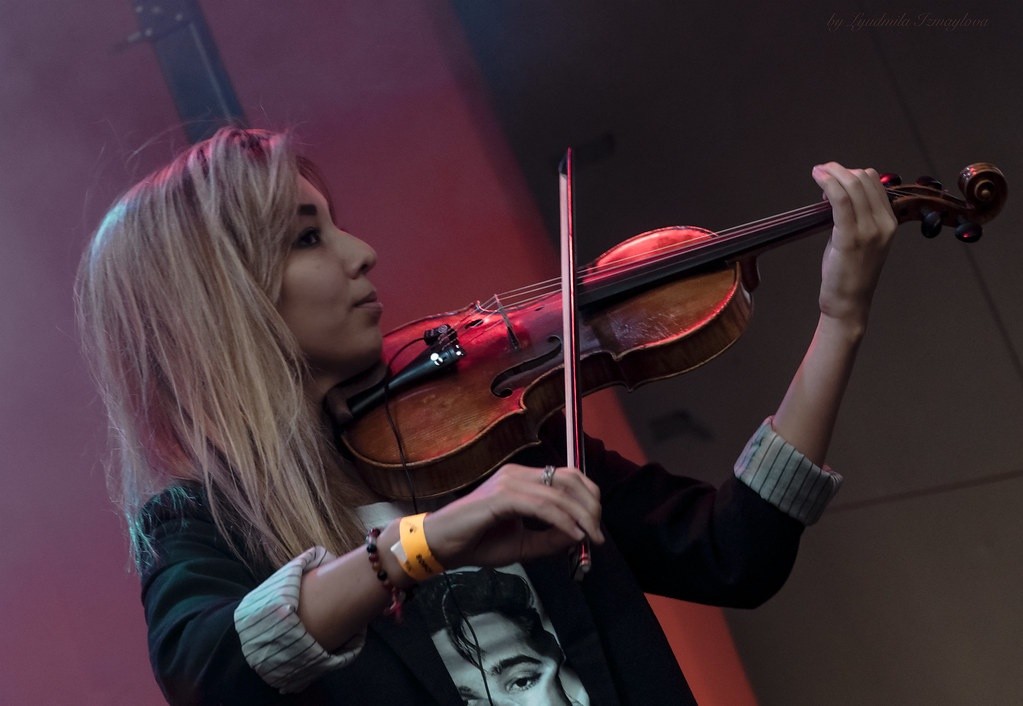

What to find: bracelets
left=390, top=511, right=447, bottom=582
left=365, top=526, right=408, bottom=621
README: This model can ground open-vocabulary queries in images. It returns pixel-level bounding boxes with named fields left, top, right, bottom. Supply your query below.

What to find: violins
left=317, top=161, right=1010, bottom=501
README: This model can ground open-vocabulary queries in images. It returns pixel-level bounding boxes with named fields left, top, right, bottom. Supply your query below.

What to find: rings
left=542, top=464, right=556, bottom=488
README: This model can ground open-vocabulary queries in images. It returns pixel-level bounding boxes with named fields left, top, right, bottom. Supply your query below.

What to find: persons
left=77, top=125, right=899, bottom=706
